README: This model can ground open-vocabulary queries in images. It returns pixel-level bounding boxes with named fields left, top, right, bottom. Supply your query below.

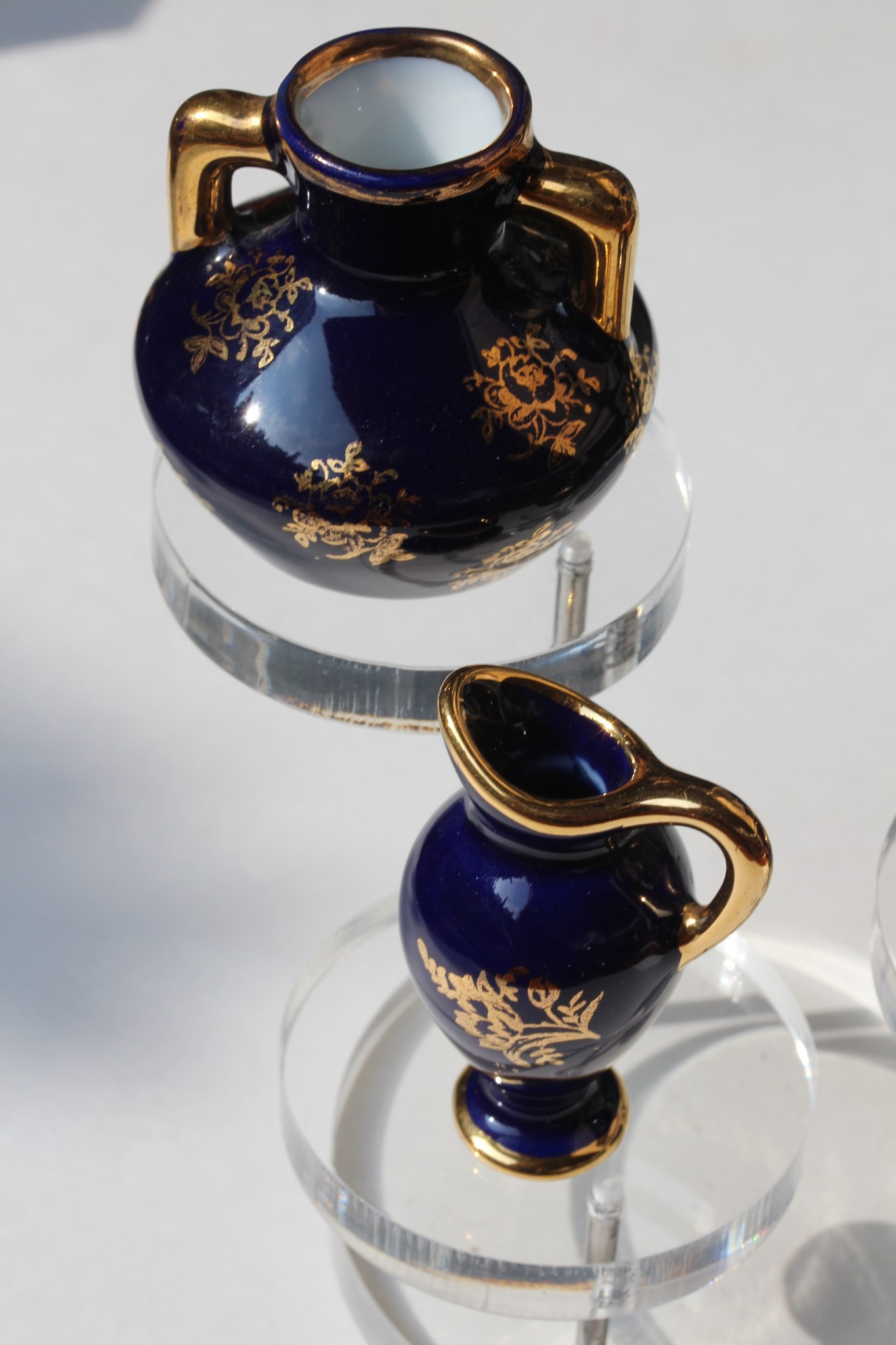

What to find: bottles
left=131, top=27, right=656, bottom=600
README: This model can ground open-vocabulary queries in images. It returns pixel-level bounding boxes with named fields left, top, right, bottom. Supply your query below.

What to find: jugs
left=397, top=664, right=776, bottom=1178
